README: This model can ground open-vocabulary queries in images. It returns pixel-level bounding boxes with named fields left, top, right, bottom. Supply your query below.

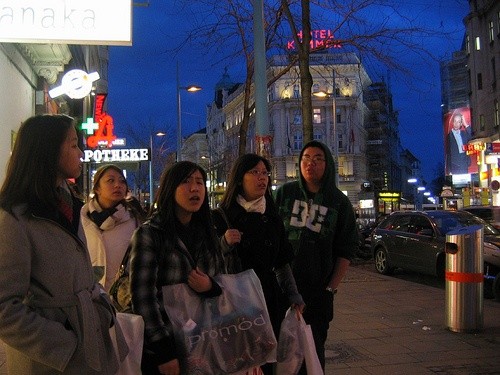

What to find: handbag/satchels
left=295, top=307, right=323, bottom=375
left=109, top=312, right=144, bottom=375
left=162, top=268, right=278, bottom=375
left=107, top=223, right=161, bottom=313
left=277, top=305, right=305, bottom=375
left=214, top=206, right=249, bottom=274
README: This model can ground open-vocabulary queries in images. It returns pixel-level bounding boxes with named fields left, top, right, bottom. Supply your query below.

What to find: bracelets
left=326, top=287, right=338, bottom=293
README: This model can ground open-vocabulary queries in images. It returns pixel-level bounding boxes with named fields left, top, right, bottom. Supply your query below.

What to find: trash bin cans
left=444, top=222, right=485, bottom=334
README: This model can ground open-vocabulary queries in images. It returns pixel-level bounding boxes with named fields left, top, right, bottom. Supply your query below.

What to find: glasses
left=301, top=157, right=326, bottom=163
left=246, top=170, right=272, bottom=176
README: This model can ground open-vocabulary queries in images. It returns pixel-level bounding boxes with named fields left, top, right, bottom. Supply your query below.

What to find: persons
left=446, top=114, right=472, bottom=174
left=126, top=160, right=227, bottom=375
left=0, top=113, right=130, bottom=375
left=78, top=164, right=138, bottom=301
left=214, top=153, right=307, bottom=375
left=274, top=141, right=357, bottom=374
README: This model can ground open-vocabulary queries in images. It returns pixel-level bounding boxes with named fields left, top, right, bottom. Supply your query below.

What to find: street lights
left=149, top=125, right=166, bottom=210
left=176, top=64, right=203, bottom=162
left=312, top=68, right=340, bottom=190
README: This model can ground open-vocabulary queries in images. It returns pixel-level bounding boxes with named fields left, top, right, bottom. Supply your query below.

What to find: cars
left=364, top=205, right=500, bottom=300
